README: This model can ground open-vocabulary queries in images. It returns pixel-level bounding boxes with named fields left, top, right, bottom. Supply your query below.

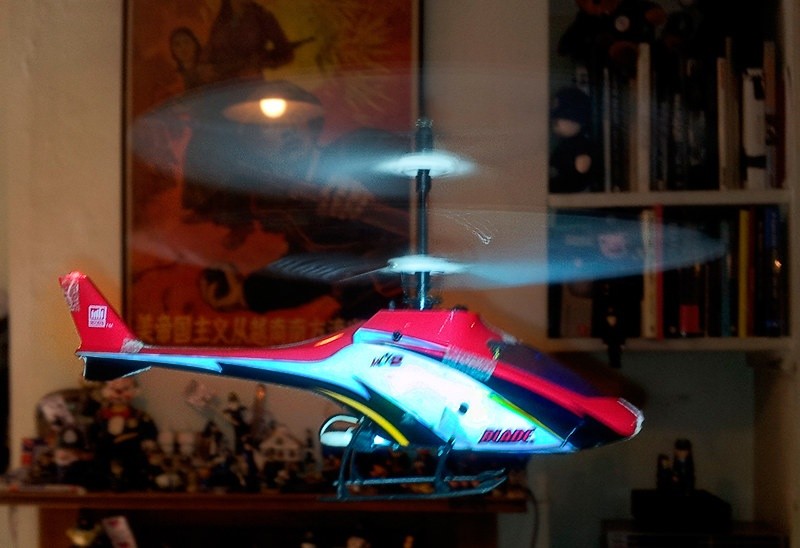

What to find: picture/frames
left=122, top=1, right=422, bottom=350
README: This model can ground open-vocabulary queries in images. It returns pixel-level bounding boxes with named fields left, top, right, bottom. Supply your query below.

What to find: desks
left=2, top=475, right=532, bottom=548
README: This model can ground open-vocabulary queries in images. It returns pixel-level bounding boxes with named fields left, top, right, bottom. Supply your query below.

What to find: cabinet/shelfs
left=543, top=0, right=800, bottom=353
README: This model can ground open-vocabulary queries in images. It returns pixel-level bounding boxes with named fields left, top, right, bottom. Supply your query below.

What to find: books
left=547, top=206, right=789, bottom=342
left=550, top=36, right=786, bottom=194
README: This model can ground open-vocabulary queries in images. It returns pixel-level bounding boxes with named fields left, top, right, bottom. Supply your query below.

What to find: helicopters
left=55, top=117, right=724, bottom=503
